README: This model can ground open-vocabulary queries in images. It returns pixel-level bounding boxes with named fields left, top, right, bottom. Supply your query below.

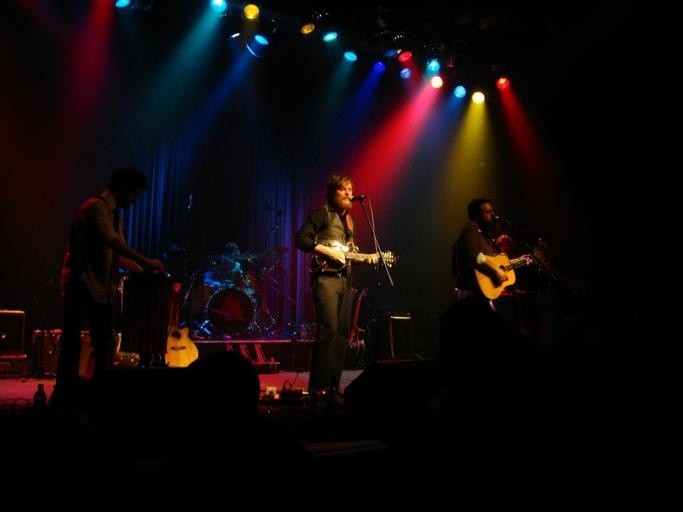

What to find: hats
left=120, top=166, right=151, bottom=193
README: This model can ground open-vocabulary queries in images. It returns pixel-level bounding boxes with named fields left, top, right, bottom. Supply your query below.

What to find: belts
left=320, top=269, right=347, bottom=277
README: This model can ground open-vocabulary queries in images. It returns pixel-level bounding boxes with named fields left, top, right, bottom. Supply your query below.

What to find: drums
left=232, top=272, right=260, bottom=299
left=346, top=287, right=371, bottom=361
left=200, top=280, right=222, bottom=314
left=193, top=284, right=256, bottom=337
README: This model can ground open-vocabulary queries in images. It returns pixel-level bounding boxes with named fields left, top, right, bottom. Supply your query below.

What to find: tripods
left=252, top=202, right=295, bottom=338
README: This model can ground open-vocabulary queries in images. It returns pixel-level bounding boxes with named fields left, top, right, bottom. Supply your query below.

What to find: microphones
left=347, top=194, right=367, bottom=201
left=495, top=215, right=512, bottom=225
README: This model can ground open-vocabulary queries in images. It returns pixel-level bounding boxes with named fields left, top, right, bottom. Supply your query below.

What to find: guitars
left=164, top=272, right=199, bottom=368
left=474, top=252, right=544, bottom=300
left=315, top=240, right=399, bottom=272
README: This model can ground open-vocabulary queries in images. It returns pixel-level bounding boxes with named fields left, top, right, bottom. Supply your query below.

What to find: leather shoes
left=313, top=388, right=347, bottom=406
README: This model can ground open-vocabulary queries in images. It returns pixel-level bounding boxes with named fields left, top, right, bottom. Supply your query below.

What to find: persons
left=55, top=165, right=165, bottom=384
left=454, top=197, right=534, bottom=304
left=293, top=174, right=398, bottom=404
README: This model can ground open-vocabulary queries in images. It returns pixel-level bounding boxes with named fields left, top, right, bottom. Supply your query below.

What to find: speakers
left=343, top=360, right=433, bottom=421
left=32, top=329, right=63, bottom=377
left=0, top=309, right=28, bottom=361
